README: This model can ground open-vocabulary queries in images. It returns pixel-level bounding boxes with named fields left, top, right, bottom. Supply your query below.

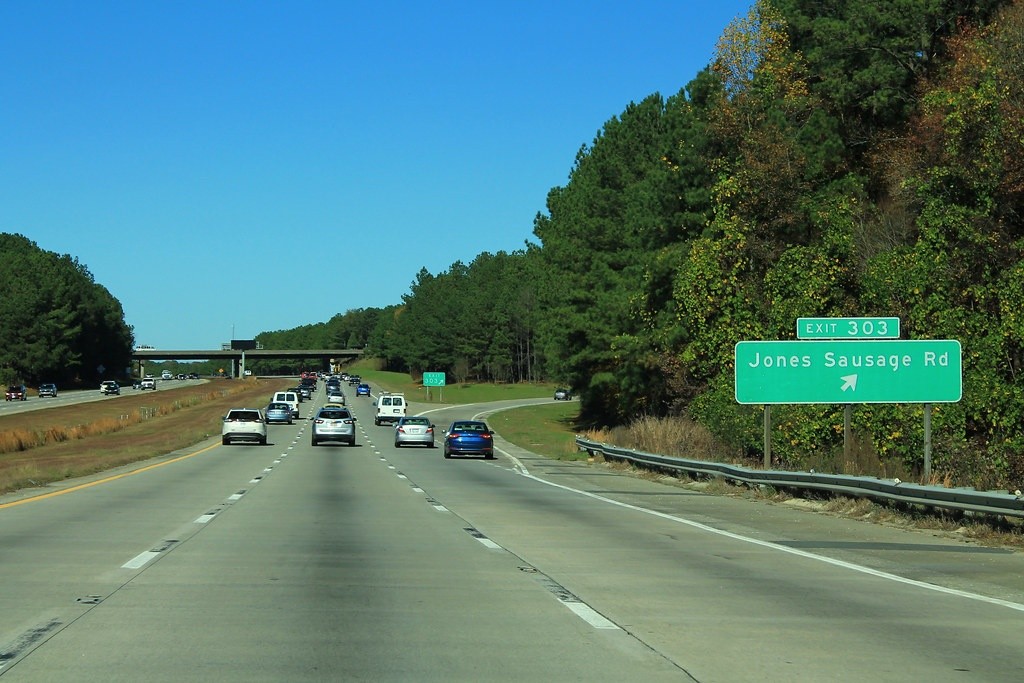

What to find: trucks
left=162, top=370, right=172, bottom=380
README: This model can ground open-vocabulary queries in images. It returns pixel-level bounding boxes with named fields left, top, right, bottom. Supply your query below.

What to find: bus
left=136, top=345, right=156, bottom=352
left=141, top=378, right=156, bottom=390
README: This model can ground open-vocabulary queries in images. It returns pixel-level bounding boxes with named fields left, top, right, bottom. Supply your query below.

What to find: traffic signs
left=733, top=341, right=965, bottom=404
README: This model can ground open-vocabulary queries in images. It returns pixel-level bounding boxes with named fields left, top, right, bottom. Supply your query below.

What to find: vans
left=271, top=391, right=300, bottom=419
left=372, top=392, right=408, bottom=426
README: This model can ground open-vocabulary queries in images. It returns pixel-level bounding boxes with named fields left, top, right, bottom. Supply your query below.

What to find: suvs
left=287, top=387, right=304, bottom=402
left=348, top=375, right=362, bottom=386
left=356, top=384, right=371, bottom=397
left=310, top=407, right=357, bottom=447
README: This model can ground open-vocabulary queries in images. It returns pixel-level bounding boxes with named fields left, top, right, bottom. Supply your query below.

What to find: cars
left=264, top=403, right=294, bottom=424
left=38, top=384, right=57, bottom=397
left=105, top=384, right=120, bottom=395
left=222, top=408, right=269, bottom=446
left=5, top=385, right=27, bottom=401
left=323, top=404, right=343, bottom=418
left=100, top=381, right=116, bottom=392
left=327, top=392, right=346, bottom=406
left=393, top=417, right=436, bottom=448
left=300, top=371, right=350, bottom=392
left=297, top=385, right=312, bottom=400
left=175, top=373, right=199, bottom=380
left=133, top=380, right=142, bottom=389
left=326, top=382, right=340, bottom=395
left=554, top=390, right=571, bottom=402
left=442, top=421, right=494, bottom=458
